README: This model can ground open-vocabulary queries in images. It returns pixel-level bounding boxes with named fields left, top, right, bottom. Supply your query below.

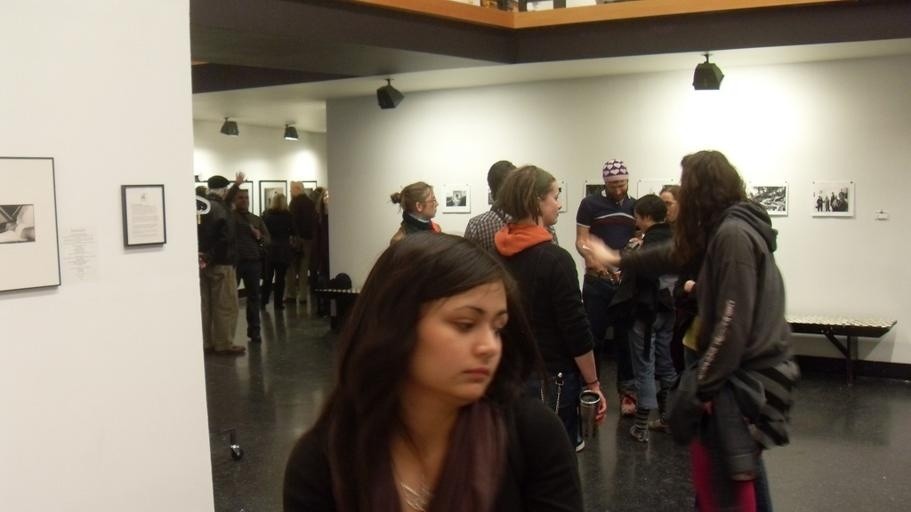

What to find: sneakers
left=628, top=424, right=650, bottom=443
left=259, top=296, right=308, bottom=310
left=617, top=390, right=637, bottom=418
left=205, top=344, right=246, bottom=355
left=649, top=418, right=670, bottom=436
left=575, top=439, right=587, bottom=452
left=247, top=327, right=262, bottom=343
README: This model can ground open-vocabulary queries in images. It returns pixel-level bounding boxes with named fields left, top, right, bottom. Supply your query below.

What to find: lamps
left=377, top=78, right=404, bottom=108
left=220, top=117, right=239, bottom=136
left=283, top=123, right=299, bottom=140
left=693, top=53, right=725, bottom=91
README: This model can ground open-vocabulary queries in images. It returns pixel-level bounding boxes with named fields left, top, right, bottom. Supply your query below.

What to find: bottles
left=578, top=389, right=602, bottom=441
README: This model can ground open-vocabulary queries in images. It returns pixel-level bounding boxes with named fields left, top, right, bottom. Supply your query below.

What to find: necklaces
left=386, top=447, right=447, bottom=512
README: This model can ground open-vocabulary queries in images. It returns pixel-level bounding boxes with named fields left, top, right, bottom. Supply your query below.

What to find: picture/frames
left=811, top=180, right=854, bottom=216
left=441, top=184, right=472, bottom=214
left=752, top=182, right=790, bottom=215
left=119, top=183, right=166, bottom=249
left=0, top=156, right=61, bottom=293
left=556, top=179, right=567, bottom=213
left=196, top=180, right=318, bottom=214
left=583, top=180, right=606, bottom=198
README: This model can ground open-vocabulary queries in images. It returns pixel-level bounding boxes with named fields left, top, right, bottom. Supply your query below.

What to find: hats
left=602, top=159, right=630, bottom=183
left=207, top=176, right=231, bottom=189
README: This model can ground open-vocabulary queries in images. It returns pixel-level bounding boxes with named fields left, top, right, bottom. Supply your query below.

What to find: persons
left=386, top=181, right=443, bottom=245
left=195, top=169, right=329, bottom=355
left=451, top=194, right=460, bottom=205
left=281, top=231, right=584, bottom=512
left=581, top=150, right=800, bottom=512
left=816, top=187, right=849, bottom=211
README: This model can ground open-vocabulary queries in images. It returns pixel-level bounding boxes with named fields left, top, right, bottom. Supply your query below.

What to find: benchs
left=787, top=313, right=898, bottom=386
left=317, top=286, right=366, bottom=332
